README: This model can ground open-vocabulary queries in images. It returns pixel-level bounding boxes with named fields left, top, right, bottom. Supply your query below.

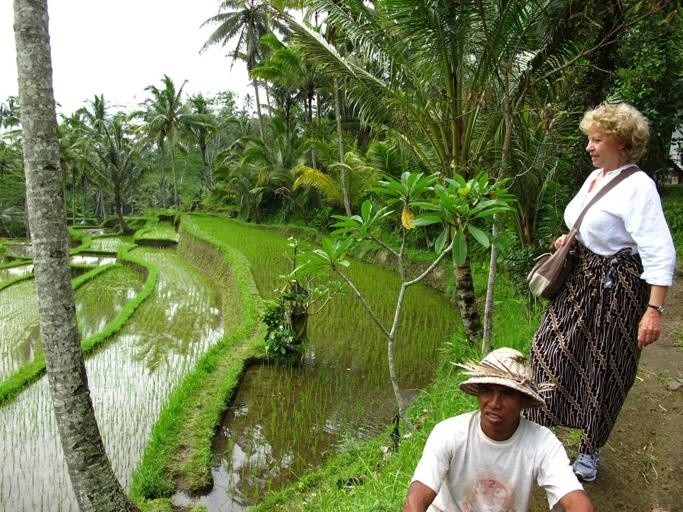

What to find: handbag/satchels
left=527, top=228, right=579, bottom=299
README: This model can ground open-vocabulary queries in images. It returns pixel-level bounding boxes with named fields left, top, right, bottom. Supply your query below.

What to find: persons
left=402, top=347, right=593, bottom=512
left=519, top=101, right=677, bottom=482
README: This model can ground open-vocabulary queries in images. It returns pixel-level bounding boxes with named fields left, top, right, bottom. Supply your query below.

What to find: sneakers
left=572, top=452, right=600, bottom=482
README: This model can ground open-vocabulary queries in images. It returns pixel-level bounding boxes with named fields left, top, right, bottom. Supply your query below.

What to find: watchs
left=647, top=304, right=665, bottom=313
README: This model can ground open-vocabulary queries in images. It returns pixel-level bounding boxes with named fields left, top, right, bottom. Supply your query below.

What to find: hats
left=458, top=347, right=547, bottom=409
